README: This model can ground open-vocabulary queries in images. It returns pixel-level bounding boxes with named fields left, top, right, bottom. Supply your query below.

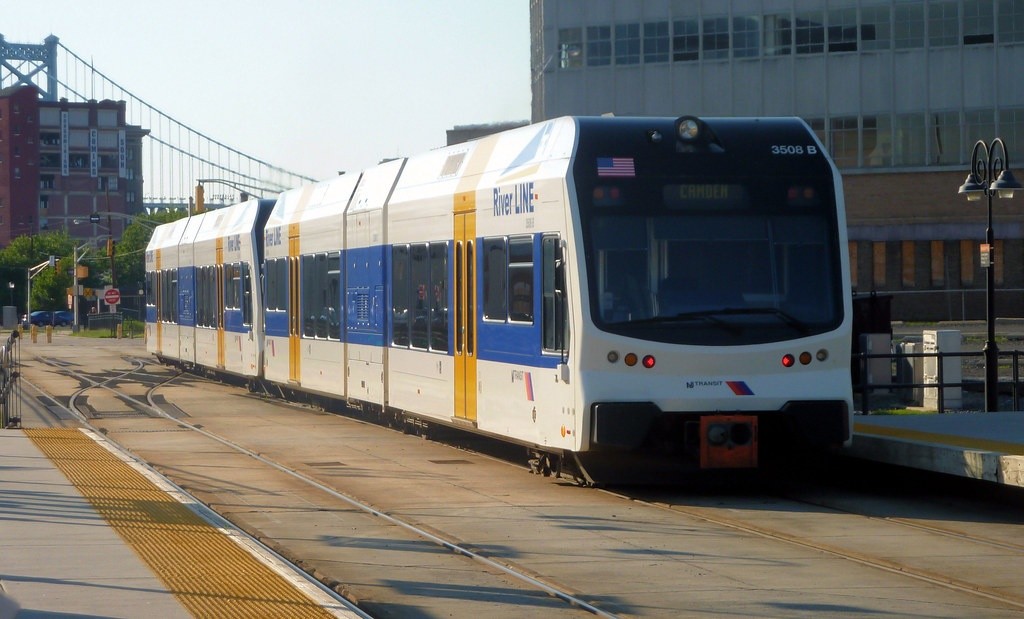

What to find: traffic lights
left=108, top=239, right=116, bottom=257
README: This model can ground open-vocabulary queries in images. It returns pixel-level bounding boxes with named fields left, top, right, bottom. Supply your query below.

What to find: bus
left=138, top=115, right=856, bottom=497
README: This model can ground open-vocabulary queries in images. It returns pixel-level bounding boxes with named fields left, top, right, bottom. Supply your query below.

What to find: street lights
left=957, top=138, right=1024, bottom=414
left=73, top=218, right=117, bottom=337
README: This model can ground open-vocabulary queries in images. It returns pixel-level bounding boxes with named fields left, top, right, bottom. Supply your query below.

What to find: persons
left=415, top=283, right=427, bottom=310
left=433, top=285, right=444, bottom=305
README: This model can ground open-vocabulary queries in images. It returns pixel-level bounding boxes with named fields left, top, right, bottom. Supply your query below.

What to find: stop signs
left=103, top=290, right=120, bottom=305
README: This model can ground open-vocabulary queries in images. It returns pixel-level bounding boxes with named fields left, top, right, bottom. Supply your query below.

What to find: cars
left=23, top=311, right=72, bottom=327
